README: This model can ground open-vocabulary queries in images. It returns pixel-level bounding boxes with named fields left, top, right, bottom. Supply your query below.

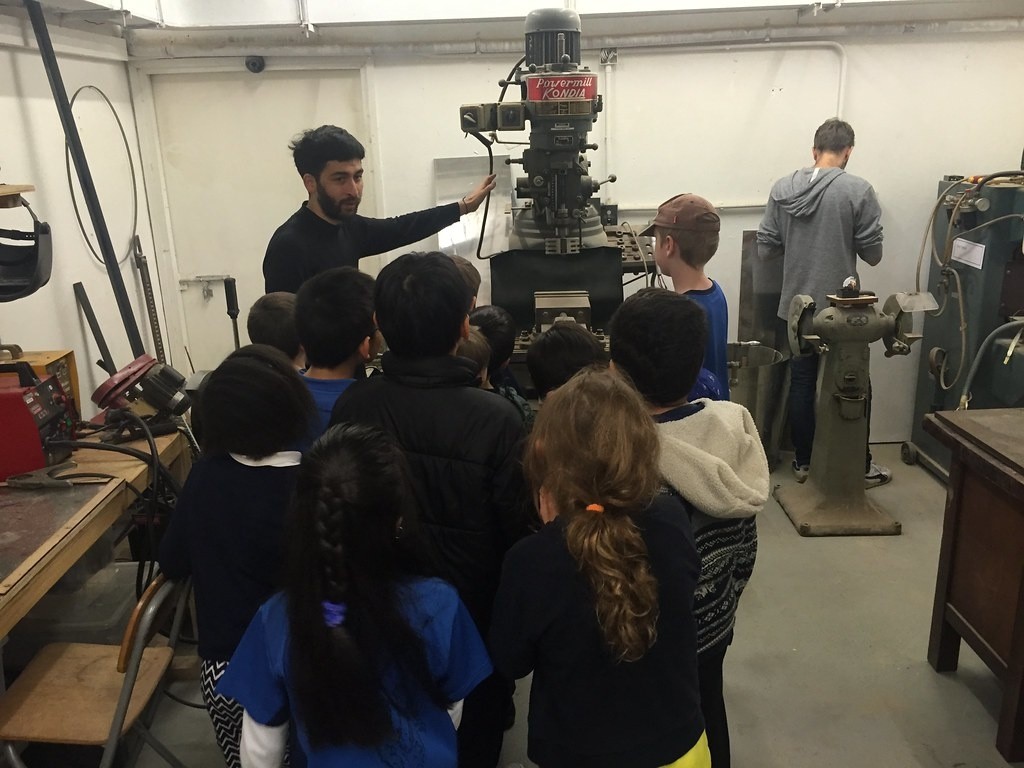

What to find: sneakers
left=865, top=462, right=892, bottom=490
left=792, top=459, right=811, bottom=483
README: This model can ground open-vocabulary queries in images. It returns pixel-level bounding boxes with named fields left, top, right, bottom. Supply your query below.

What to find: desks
left=921, top=408, right=1024, bottom=763
left=0, top=403, right=191, bottom=639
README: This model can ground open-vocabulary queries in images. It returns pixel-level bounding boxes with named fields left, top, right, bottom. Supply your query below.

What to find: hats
left=637, top=192, right=721, bottom=237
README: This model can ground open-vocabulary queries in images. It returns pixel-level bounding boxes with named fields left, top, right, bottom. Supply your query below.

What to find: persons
left=157, top=125, right=770, bottom=767
left=756, top=116, right=892, bottom=490
left=214, top=422, right=492, bottom=768
left=329, top=251, right=545, bottom=768
left=486, top=363, right=713, bottom=767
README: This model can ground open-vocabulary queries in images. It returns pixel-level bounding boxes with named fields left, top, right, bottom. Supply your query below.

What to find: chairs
left=0, top=572, right=191, bottom=768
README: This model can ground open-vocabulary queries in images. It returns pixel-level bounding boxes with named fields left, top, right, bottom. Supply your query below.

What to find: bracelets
left=462, top=196, right=468, bottom=214
